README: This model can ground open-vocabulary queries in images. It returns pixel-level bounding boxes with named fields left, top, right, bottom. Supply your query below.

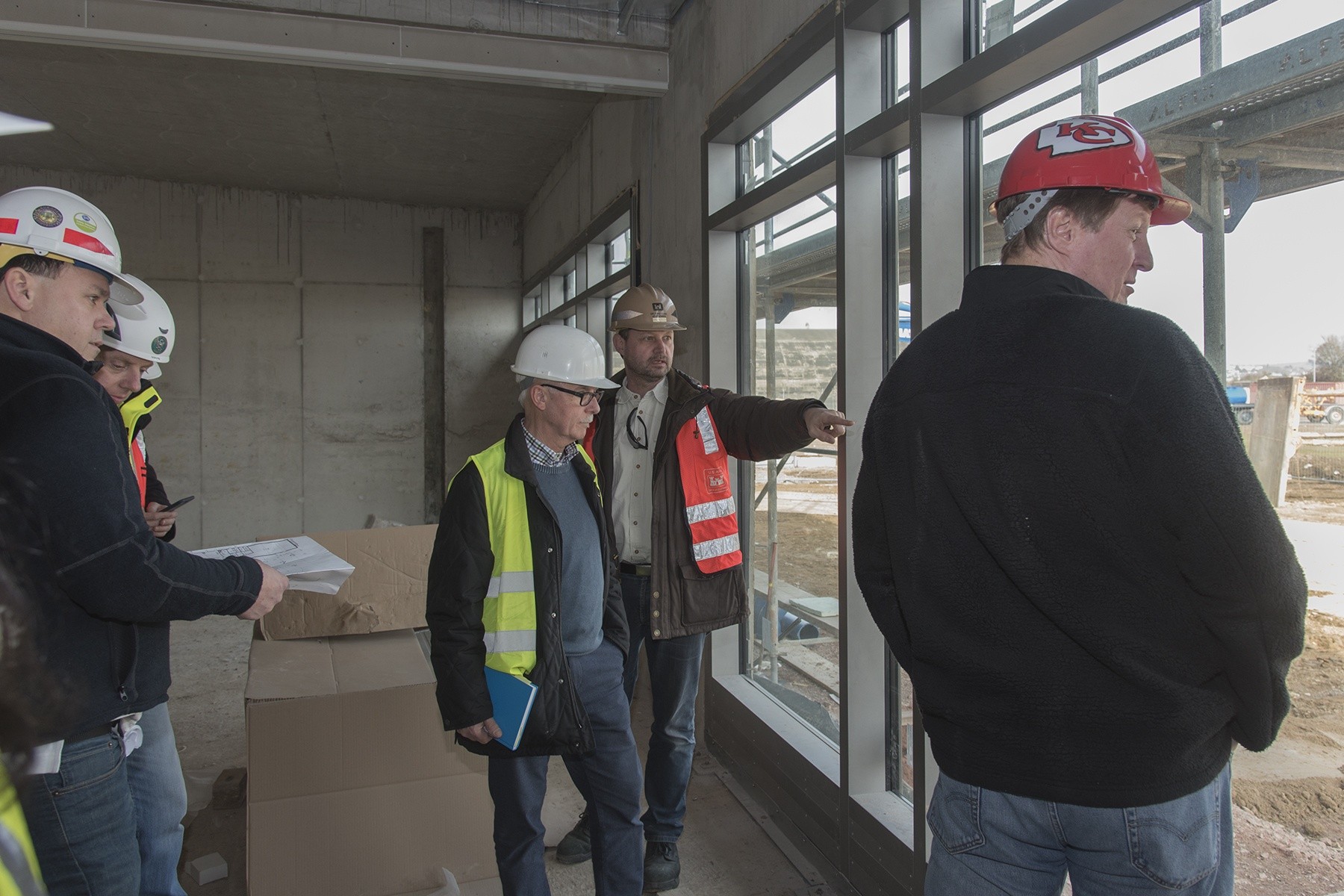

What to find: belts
left=619, top=562, right=651, bottom=576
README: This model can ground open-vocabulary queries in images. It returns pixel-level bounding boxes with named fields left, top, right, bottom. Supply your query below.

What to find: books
left=482, top=664, right=539, bottom=750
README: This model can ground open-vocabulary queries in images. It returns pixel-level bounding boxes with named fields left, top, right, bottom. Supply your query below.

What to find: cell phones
left=158, top=495, right=195, bottom=513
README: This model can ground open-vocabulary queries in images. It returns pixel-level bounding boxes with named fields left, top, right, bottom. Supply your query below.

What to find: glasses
left=626, top=407, right=649, bottom=450
left=541, top=383, right=605, bottom=407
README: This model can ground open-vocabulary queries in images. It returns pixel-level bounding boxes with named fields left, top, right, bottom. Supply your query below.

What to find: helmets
left=0, top=187, right=144, bottom=306
left=100, top=273, right=175, bottom=363
left=510, top=325, right=621, bottom=389
left=142, top=362, right=163, bottom=380
left=988, top=116, right=1192, bottom=225
left=607, top=283, right=687, bottom=331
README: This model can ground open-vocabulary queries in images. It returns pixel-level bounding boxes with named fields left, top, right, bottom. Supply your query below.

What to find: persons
left=83, top=273, right=189, bottom=896
left=553, top=285, right=855, bottom=893
left=849, top=116, right=1308, bottom=896
left=425, top=325, right=645, bottom=896
left=1, top=186, right=289, bottom=896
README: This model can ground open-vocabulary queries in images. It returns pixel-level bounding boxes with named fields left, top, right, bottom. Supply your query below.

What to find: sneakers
left=556, top=809, right=594, bottom=863
left=643, top=841, right=681, bottom=892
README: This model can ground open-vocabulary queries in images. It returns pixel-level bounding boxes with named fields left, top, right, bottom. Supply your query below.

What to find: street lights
left=1313, top=347, right=1318, bottom=382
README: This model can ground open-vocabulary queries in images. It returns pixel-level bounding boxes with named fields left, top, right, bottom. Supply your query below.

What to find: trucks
left=1224, top=382, right=1344, bottom=425
